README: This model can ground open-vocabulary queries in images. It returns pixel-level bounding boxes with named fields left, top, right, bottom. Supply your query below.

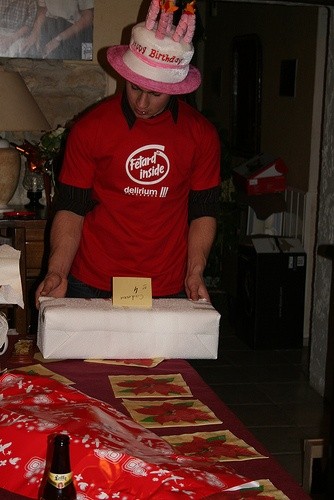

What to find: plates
left=4, top=212, right=36, bottom=219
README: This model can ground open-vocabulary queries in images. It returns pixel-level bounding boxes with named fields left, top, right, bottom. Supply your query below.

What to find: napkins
left=160, top=430, right=269, bottom=463
left=120, top=399, right=223, bottom=428
left=5, top=364, right=76, bottom=386
left=230, top=478, right=290, bottom=500
left=84, top=357, right=165, bottom=369
left=107, top=373, right=193, bottom=398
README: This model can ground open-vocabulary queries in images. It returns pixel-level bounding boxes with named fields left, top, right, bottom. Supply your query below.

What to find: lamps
left=0, top=71, right=53, bottom=212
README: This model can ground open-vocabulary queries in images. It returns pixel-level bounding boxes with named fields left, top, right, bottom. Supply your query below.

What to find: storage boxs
left=233, top=154, right=288, bottom=195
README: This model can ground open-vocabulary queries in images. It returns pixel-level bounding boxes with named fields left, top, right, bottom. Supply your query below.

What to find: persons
left=35, top=0, right=222, bottom=310
left=0, top=0, right=94, bottom=60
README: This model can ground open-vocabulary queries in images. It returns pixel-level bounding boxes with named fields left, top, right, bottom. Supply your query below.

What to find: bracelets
left=55, top=34, right=63, bottom=47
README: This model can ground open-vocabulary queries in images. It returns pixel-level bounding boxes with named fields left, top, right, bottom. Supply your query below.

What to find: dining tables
left=0, top=328, right=314, bottom=500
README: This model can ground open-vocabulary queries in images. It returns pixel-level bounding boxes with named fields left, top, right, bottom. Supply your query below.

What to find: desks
left=0, top=211, right=49, bottom=333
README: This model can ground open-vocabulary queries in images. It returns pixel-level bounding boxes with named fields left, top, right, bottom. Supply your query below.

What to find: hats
left=105, top=0, right=202, bottom=96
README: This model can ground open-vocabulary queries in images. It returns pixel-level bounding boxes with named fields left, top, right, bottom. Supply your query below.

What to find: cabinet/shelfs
left=237, top=242, right=307, bottom=350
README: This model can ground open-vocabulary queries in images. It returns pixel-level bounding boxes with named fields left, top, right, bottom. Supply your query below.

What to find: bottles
left=43, top=434, right=76, bottom=500
